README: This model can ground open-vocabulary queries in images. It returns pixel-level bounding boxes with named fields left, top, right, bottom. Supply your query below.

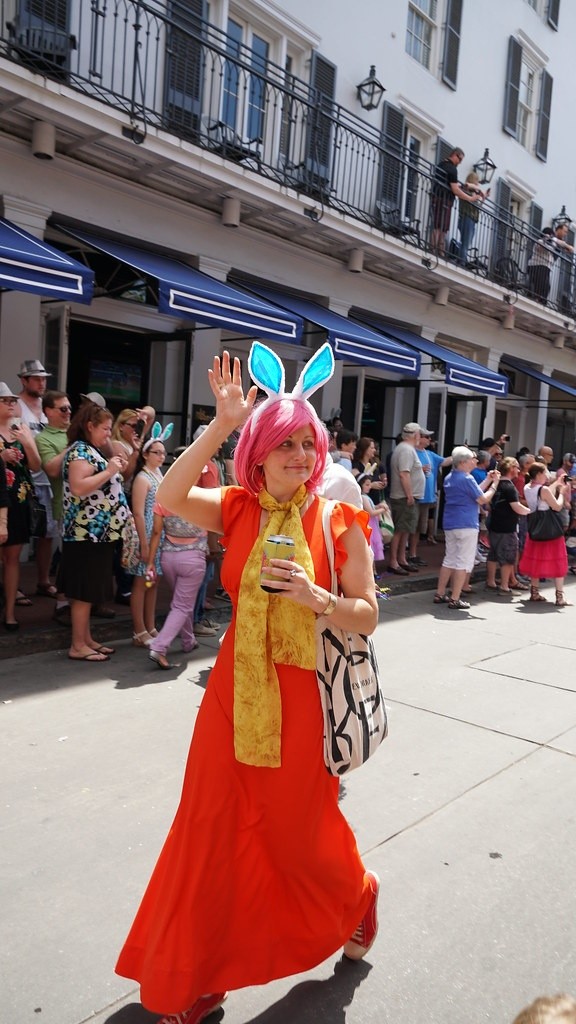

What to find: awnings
left=220, top=277, right=422, bottom=377
left=351, top=314, right=509, bottom=403
left=499, top=360, right=576, bottom=411
left=0, top=215, right=94, bottom=307
left=40, top=218, right=305, bottom=346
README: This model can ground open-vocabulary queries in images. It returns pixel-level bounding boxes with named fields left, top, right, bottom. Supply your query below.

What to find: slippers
left=95, top=645, right=115, bottom=654
left=69, top=651, right=110, bottom=661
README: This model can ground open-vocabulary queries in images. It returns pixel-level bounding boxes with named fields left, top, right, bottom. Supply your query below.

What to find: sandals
left=132, top=585, right=232, bottom=670
left=387, top=534, right=576, bottom=609
left=36, top=583, right=62, bottom=600
left=15, top=589, right=34, bottom=606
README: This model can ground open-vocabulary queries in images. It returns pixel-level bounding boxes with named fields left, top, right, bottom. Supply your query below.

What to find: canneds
left=267, top=534, right=295, bottom=545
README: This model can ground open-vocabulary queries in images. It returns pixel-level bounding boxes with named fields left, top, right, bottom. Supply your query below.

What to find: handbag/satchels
left=527, top=487, right=564, bottom=541
left=0, top=507, right=7, bottom=544
left=315, top=500, right=388, bottom=777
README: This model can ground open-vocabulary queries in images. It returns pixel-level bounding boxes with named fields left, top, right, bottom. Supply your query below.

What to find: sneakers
left=344, top=868, right=380, bottom=961
left=158, top=988, right=228, bottom=1024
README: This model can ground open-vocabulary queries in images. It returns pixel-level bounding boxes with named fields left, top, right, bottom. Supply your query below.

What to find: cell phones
left=135, top=419, right=145, bottom=439
left=505, top=436, right=511, bottom=441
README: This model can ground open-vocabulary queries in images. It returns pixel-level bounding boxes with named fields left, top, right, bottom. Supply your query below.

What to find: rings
left=290, top=570, right=296, bottom=577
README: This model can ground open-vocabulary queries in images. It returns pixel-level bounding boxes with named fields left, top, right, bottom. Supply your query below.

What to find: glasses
left=0, top=399, right=18, bottom=406
left=123, top=422, right=137, bottom=428
left=147, top=450, right=167, bottom=456
left=515, top=466, right=521, bottom=470
left=56, top=406, right=72, bottom=412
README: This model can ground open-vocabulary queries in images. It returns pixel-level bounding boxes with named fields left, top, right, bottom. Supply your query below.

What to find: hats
left=419, top=427, right=433, bottom=436
left=0, top=383, right=19, bottom=397
left=404, top=423, right=419, bottom=434
left=17, top=360, right=51, bottom=377
left=81, top=393, right=105, bottom=408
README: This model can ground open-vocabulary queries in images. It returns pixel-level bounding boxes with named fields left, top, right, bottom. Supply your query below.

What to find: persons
left=456, top=171, right=490, bottom=267
left=517, top=447, right=576, bottom=606
left=548, top=223, right=576, bottom=297
left=385, top=422, right=454, bottom=576
left=429, top=147, right=479, bottom=261
left=323, top=405, right=387, bottom=581
left=130, top=406, right=254, bottom=669
left=527, top=227, right=567, bottom=307
left=433, top=433, right=529, bottom=609
left=115, top=332, right=379, bottom=1024
left=0, top=359, right=158, bottom=663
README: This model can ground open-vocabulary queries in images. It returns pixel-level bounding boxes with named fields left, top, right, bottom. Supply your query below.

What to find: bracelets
left=490, top=486, right=497, bottom=492
left=495, top=443, right=501, bottom=447
left=317, top=593, right=337, bottom=616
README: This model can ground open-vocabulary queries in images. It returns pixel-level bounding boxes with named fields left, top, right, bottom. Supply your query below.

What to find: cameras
left=564, top=476, right=573, bottom=484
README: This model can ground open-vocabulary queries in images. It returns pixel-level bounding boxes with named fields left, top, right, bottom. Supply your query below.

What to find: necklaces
left=231, top=433, right=237, bottom=441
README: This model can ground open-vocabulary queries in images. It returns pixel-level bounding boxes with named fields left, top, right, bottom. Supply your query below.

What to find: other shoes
left=91, top=606, right=116, bottom=619
left=115, top=593, right=132, bottom=607
left=52, top=606, right=72, bottom=624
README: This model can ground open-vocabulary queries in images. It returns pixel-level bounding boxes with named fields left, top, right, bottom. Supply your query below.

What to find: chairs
left=6, top=21, right=78, bottom=82
left=449, top=237, right=488, bottom=277
left=377, top=206, right=423, bottom=248
left=207, top=121, right=263, bottom=171
left=496, top=257, right=530, bottom=292
left=557, top=289, right=576, bottom=321
left=283, top=160, right=338, bottom=206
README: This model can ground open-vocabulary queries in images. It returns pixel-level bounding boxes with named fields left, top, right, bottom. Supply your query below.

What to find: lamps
left=356, top=65, right=386, bottom=109
left=348, top=248, right=364, bottom=274
left=434, top=286, right=449, bottom=306
left=504, top=314, right=514, bottom=329
left=32, top=119, right=55, bottom=159
left=221, top=198, right=241, bottom=227
left=552, top=205, right=572, bottom=237
left=553, top=336, right=565, bottom=348
left=472, top=147, right=498, bottom=184
